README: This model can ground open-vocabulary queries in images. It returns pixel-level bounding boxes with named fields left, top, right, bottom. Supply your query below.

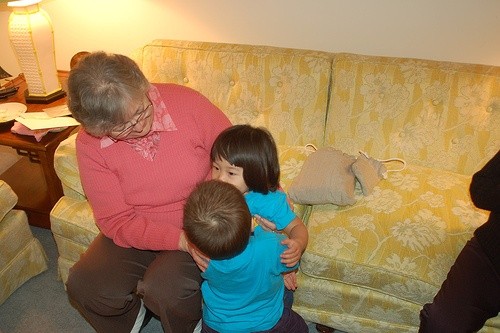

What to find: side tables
left=0, top=69, right=80, bottom=231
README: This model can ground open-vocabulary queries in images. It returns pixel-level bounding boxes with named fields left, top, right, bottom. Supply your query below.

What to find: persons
left=181, top=178, right=310, bottom=333
left=65, top=52, right=293, bottom=332
left=210, top=124, right=309, bottom=268
left=417, top=149, right=500, bottom=333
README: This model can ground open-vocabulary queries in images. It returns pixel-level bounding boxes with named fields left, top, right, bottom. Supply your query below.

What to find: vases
left=6, top=0, right=68, bottom=105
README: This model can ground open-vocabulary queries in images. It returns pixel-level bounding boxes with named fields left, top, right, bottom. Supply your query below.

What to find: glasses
left=108, top=95, right=153, bottom=143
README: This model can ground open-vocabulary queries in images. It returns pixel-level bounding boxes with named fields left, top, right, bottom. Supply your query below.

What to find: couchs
left=0, top=38, right=500, bottom=333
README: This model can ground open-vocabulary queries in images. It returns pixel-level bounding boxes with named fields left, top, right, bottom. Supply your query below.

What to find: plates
left=0, top=102, right=27, bottom=123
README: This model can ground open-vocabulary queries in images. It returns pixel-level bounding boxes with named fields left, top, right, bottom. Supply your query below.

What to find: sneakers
left=193, top=318, right=202, bottom=333
left=129, top=299, right=147, bottom=333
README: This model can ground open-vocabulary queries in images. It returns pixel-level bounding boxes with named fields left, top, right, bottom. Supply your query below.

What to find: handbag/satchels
left=287, top=143, right=356, bottom=206
left=351, top=150, right=406, bottom=196
